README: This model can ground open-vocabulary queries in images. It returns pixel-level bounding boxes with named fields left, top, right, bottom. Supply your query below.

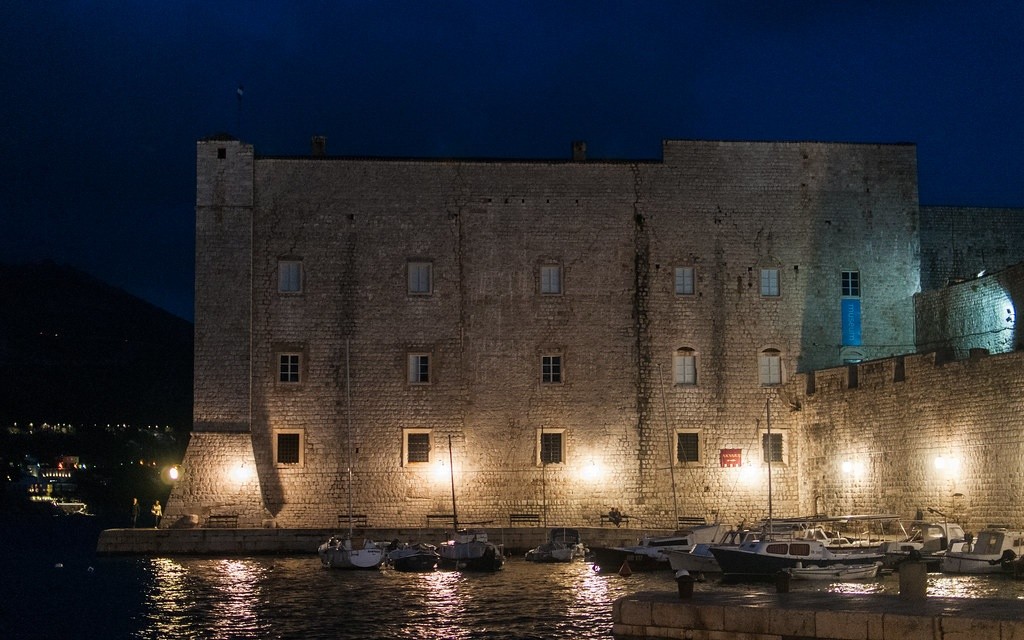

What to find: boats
left=658, top=514, right=909, bottom=577
left=940, top=528, right=1024, bottom=579
left=790, top=562, right=879, bottom=579
left=877, top=520, right=978, bottom=569
left=387, top=538, right=440, bottom=571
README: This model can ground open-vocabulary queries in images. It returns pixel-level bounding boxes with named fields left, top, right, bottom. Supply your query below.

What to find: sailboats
left=524, top=425, right=575, bottom=562
left=435, top=434, right=507, bottom=570
left=317, top=338, right=387, bottom=569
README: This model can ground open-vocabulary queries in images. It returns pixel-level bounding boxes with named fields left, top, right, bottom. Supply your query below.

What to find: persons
left=151, top=500, right=162, bottom=529
left=127, top=498, right=140, bottom=528
left=388, top=539, right=401, bottom=552
left=609, top=508, right=622, bottom=528
left=737, top=525, right=744, bottom=543
left=484, top=548, right=495, bottom=565
